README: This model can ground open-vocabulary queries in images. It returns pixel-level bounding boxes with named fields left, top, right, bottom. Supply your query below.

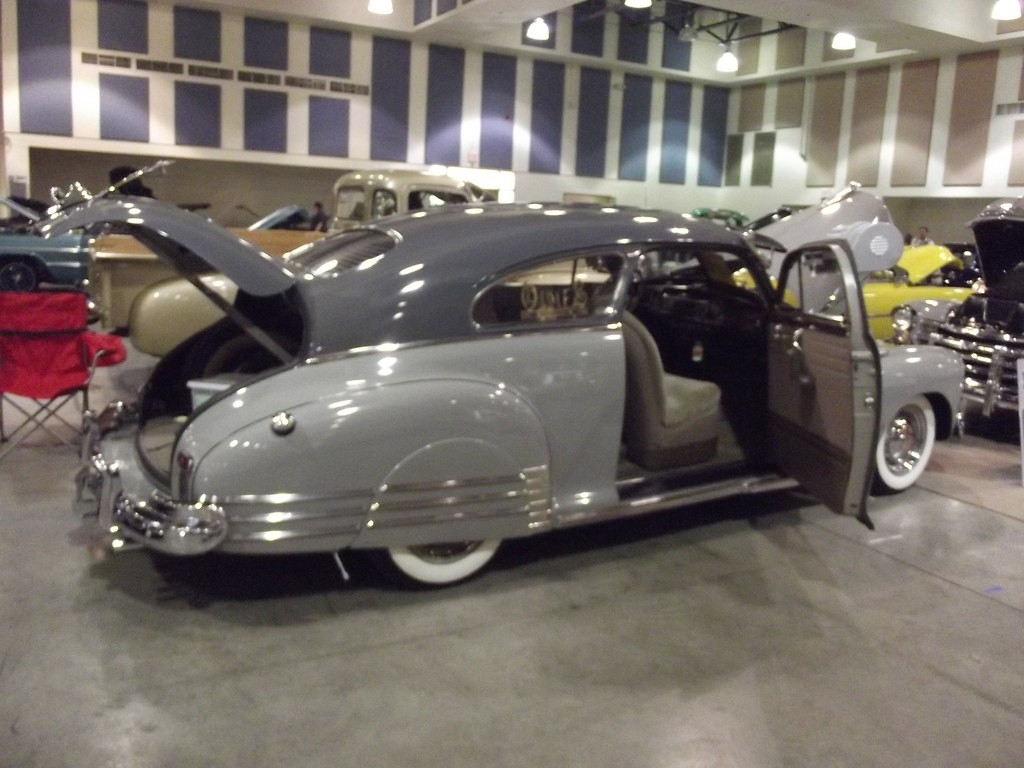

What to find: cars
left=30, top=156, right=972, bottom=596
left=1, top=196, right=108, bottom=295
left=885, top=194, right=1023, bottom=437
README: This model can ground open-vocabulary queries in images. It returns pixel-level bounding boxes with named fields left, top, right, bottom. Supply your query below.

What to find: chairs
left=0, top=292, right=128, bottom=459
left=595, top=300, right=722, bottom=471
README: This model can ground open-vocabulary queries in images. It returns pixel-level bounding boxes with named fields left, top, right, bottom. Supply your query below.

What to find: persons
left=109, top=165, right=154, bottom=198
left=310, top=202, right=327, bottom=232
left=911, top=227, right=934, bottom=246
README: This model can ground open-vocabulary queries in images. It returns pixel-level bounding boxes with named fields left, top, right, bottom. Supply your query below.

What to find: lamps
left=527, top=15, right=549, bottom=40
left=718, top=42, right=739, bottom=71
left=678, top=12, right=698, bottom=40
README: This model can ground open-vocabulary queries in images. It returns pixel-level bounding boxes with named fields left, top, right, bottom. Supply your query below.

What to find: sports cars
left=730, top=235, right=980, bottom=355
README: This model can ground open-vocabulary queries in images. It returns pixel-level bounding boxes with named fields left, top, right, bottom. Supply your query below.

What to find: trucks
left=88, top=167, right=490, bottom=333
